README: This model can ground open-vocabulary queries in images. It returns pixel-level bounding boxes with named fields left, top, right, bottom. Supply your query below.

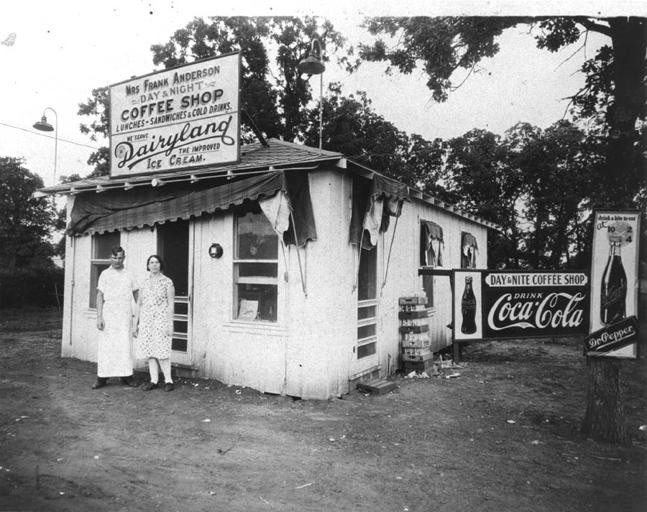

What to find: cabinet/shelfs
left=601, top=237, right=627, bottom=324
left=461, top=277, right=476, bottom=334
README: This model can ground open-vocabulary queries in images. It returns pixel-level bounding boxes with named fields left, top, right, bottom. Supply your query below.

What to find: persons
left=133, top=255, right=175, bottom=391
left=92, top=246, right=139, bottom=389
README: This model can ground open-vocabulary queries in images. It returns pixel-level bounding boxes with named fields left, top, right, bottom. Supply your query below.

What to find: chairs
left=143, top=383, right=158, bottom=390
left=164, top=383, right=173, bottom=392
left=123, top=377, right=139, bottom=387
left=92, top=377, right=105, bottom=389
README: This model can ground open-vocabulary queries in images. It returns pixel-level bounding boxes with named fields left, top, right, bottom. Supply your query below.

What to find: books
left=398, top=296, right=434, bottom=373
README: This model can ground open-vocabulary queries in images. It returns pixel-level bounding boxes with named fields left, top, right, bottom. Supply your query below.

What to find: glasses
left=33, top=107, right=57, bottom=186
left=299, top=38, right=325, bottom=157
